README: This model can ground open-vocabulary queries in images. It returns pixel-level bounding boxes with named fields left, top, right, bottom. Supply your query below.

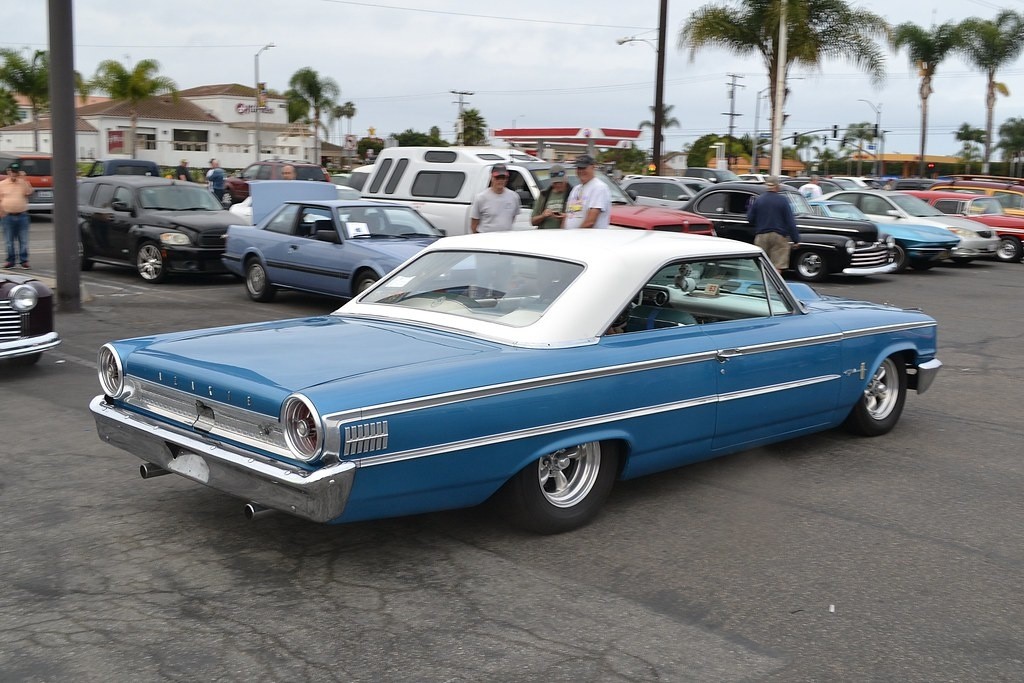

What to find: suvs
left=618, top=174, right=716, bottom=210
left=74, top=174, right=251, bottom=283
left=0, top=149, right=53, bottom=216
left=222, top=160, right=330, bottom=207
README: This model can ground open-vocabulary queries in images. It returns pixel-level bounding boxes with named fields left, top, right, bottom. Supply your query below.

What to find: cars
left=331, top=163, right=373, bottom=194
left=220, top=179, right=478, bottom=300
left=681, top=180, right=898, bottom=282
left=1, top=270, right=61, bottom=372
left=806, top=189, right=1001, bottom=268
left=88, top=225, right=944, bottom=536
left=86, top=157, right=160, bottom=199
left=678, top=166, right=1024, bottom=220
left=898, top=190, right=1023, bottom=262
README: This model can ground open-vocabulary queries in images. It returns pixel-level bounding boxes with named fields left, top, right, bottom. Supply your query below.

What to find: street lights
left=858, top=98, right=881, bottom=173
left=752, top=76, right=806, bottom=174
left=254, top=42, right=276, bottom=161
left=617, top=38, right=665, bottom=173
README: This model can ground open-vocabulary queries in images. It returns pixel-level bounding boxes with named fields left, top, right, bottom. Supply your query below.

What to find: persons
left=0, top=162, right=34, bottom=270
left=747, top=175, right=801, bottom=275
left=322, top=158, right=338, bottom=175
left=799, top=174, right=823, bottom=197
left=281, top=164, right=297, bottom=180
left=530, top=165, right=574, bottom=294
left=563, top=154, right=611, bottom=229
left=177, top=159, right=226, bottom=202
left=471, top=164, right=522, bottom=299
left=882, top=179, right=895, bottom=190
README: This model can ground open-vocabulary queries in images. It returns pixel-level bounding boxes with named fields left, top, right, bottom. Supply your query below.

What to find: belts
left=9, top=211, right=28, bottom=216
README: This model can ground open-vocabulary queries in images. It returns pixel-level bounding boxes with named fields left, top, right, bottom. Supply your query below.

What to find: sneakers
left=21, top=262, right=30, bottom=270
left=4, top=261, right=16, bottom=270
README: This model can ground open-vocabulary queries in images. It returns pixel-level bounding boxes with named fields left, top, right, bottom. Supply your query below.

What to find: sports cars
left=788, top=201, right=964, bottom=272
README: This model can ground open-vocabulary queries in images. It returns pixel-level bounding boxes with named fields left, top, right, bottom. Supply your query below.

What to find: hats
left=11, top=163, right=20, bottom=172
left=573, top=156, right=593, bottom=170
left=492, top=164, right=509, bottom=178
left=766, top=176, right=779, bottom=188
left=549, top=166, right=564, bottom=183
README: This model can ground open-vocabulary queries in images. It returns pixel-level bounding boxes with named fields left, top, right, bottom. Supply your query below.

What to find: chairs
left=308, top=219, right=333, bottom=245
left=627, top=305, right=697, bottom=333
left=365, top=213, right=394, bottom=234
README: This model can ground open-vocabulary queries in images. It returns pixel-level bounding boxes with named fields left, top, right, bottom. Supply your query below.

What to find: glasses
left=551, top=172, right=564, bottom=177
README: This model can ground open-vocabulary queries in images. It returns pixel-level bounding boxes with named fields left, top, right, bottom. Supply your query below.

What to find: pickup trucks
left=361, top=145, right=715, bottom=269
